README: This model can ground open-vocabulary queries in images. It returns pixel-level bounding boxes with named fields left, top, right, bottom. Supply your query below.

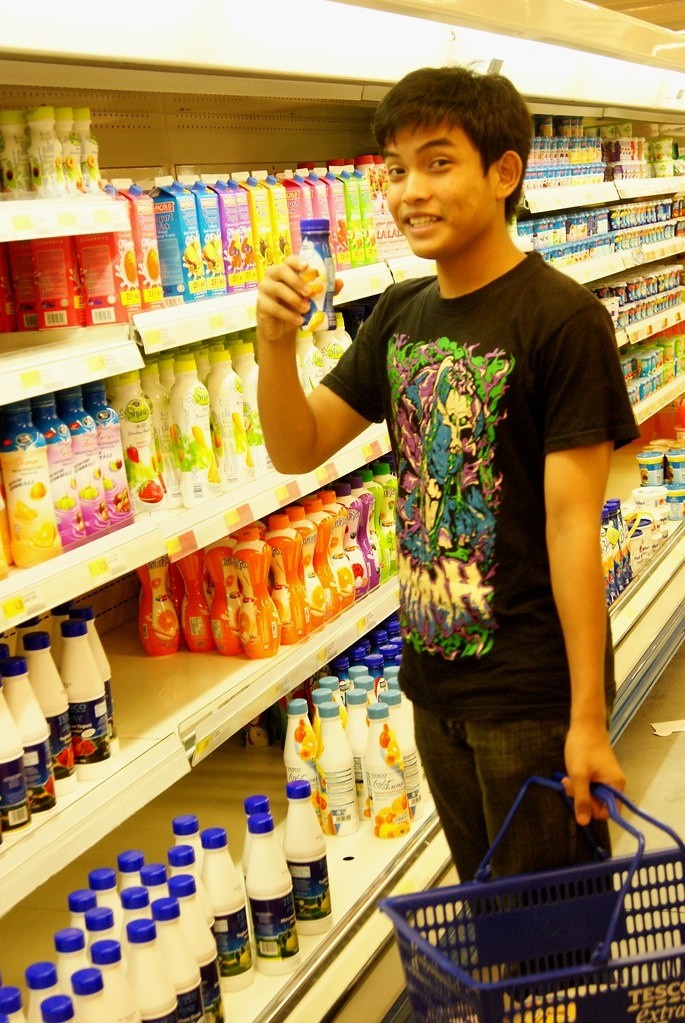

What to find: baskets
left=378, top=769, right=685, bottom=1023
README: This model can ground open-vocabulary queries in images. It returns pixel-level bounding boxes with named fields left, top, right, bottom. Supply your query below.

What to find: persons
left=256, top=67, right=640, bottom=1023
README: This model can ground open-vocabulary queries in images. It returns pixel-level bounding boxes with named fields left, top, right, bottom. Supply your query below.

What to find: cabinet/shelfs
left=1, top=44, right=684, bottom=1023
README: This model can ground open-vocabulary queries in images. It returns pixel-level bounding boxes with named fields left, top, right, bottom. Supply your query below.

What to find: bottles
left=0, top=107, right=634, bottom=1022
